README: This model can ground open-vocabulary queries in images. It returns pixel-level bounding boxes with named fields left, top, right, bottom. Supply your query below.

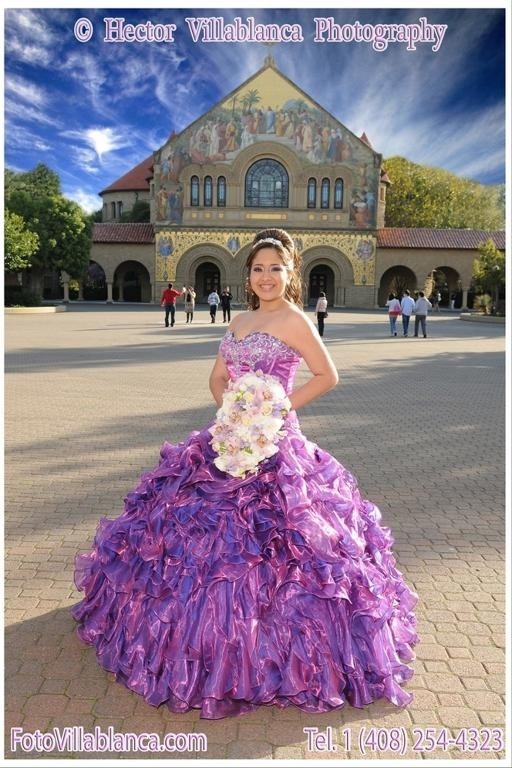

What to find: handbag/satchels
left=394, top=300, right=401, bottom=313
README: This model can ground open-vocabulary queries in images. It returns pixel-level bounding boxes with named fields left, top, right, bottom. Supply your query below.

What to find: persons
left=415, top=291, right=432, bottom=338
left=314, top=291, right=328, bottom=336
left=154, top=103, right=379, bottom=229
left=387, top=292, right=401, bottom=336
left=69, top=227, right=422, bottom=720
left=219, top=288, right=233, bottom=324
left=207, top=289, right=220, bottom=323
left=400, top=289, right=416, bottom=337
left=160, top=283, right=186, bottom=327
left=183, top=286, right=196, bottom=324
left=433, top=290, right=458, bottom=313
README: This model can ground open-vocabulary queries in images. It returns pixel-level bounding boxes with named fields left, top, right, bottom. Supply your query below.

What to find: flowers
left=208, top=371, right=291, bottom=480
